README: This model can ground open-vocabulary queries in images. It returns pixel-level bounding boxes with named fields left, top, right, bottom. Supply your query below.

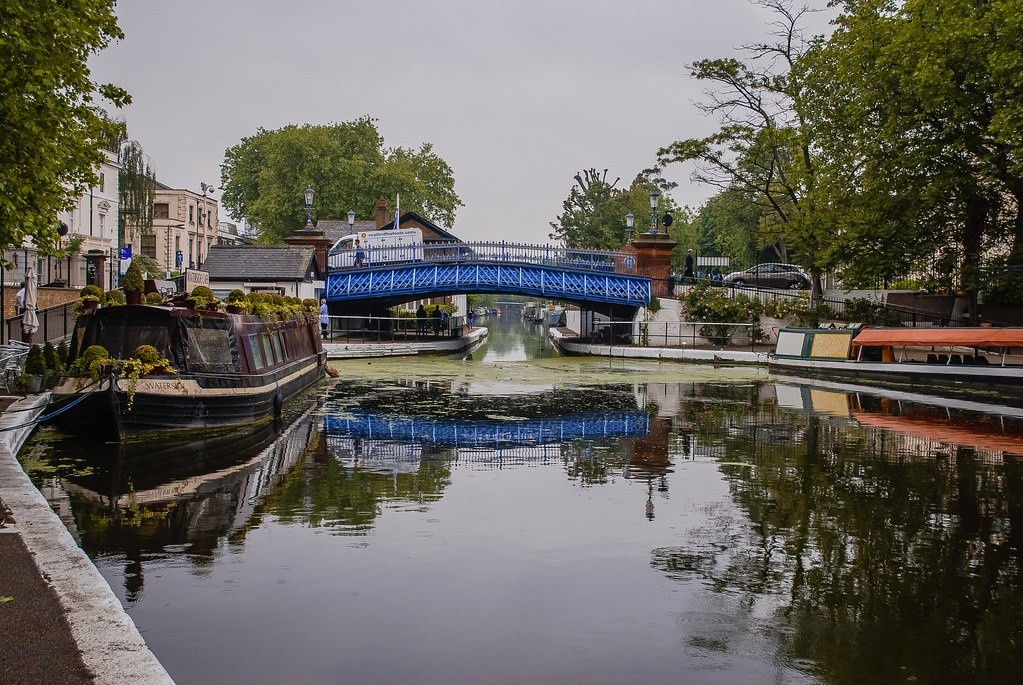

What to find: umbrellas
left=23, top=267, right=40, bottom=349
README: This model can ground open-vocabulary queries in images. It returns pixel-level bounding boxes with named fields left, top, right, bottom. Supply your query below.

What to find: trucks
left=326, top=228, right=424, bottom=269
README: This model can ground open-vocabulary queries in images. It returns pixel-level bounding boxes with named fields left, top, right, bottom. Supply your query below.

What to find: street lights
left=626, top=212, right=634, bottom=246
left=347, top=210, right=355, bottom=234
left=649, top=188, right=658, bottom=233
left=202, top=181, right=214, bottom=262
left=305, top=185, right=314, bottom=230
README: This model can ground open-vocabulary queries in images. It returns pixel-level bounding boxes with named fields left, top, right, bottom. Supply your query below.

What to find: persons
left=320, top=299, right=329, bottom=340
left=467, top=305, right=474, bottom=330
left=680, top=249, right=695, bottom=283
left=353, top=239, right=365, bottom=268
left=14, top=281, right=31, bottom=343
left=432, top=305, right=442, bottom=336
left=416, top=304, right=428, bottom=335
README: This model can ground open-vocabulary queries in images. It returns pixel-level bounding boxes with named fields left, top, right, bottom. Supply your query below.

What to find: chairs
left=0, top=339, right=43, bottom=395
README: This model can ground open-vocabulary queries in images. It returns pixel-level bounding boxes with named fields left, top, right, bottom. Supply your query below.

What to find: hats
left=688, top=249, right=693, bottom=252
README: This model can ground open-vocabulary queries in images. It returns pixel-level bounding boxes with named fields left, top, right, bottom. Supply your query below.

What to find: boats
left=769, top=367, right=1023, bottom=458
left=50, top=291, right=328, bottom=427
left=767, top=321, right=1023, bottom=380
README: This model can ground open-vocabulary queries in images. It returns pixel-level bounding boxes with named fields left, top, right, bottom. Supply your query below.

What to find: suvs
left=722, top=262, right=812, bottom=290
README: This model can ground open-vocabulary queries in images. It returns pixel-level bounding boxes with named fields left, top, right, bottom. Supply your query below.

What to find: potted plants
left=22, top=344, right=46, bottom=394
left=72, top=261, right=246, bottom=314
